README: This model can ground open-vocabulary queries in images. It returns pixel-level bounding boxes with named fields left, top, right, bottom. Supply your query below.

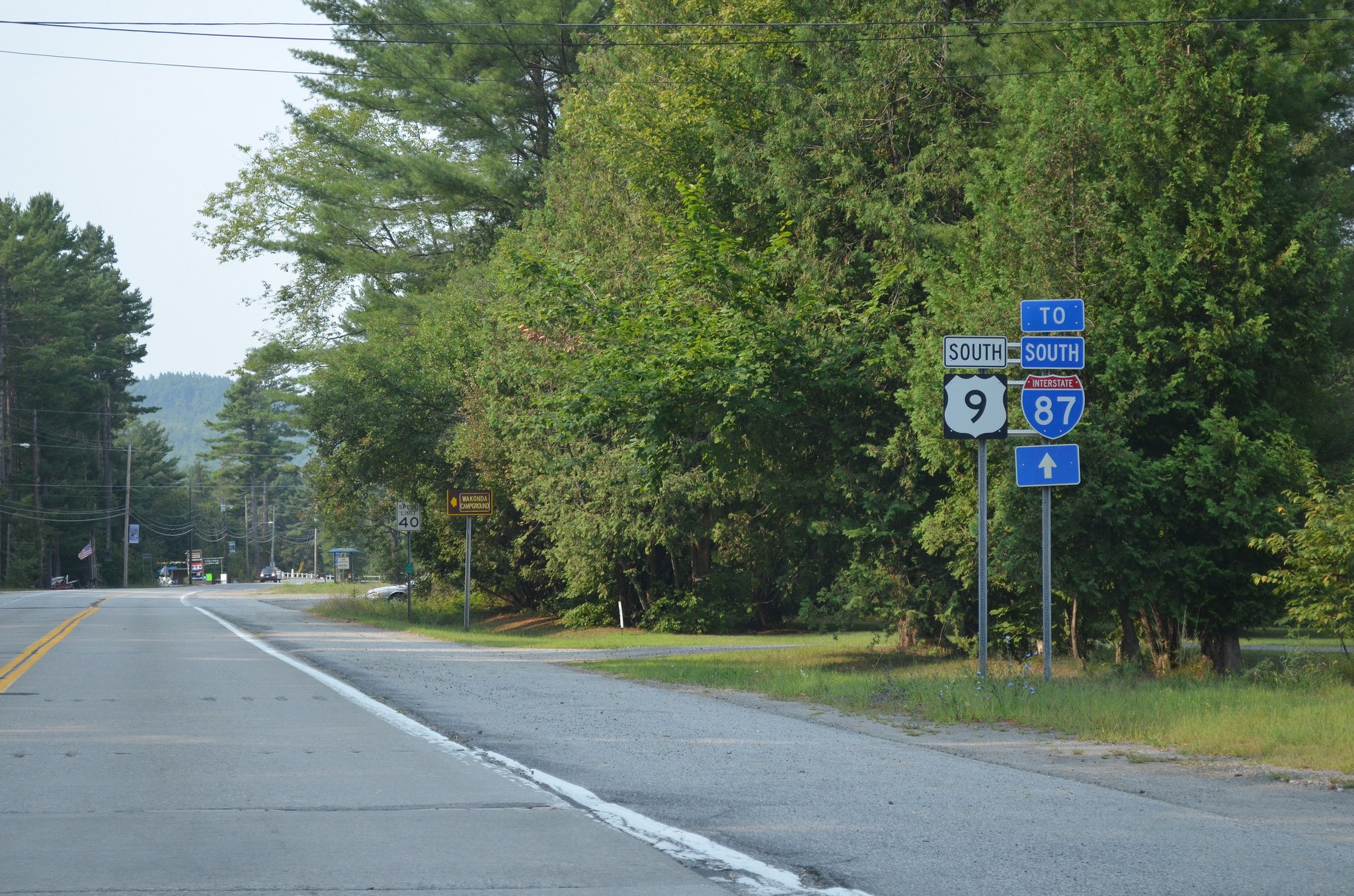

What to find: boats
left=51, top=576, right=65, bottom=586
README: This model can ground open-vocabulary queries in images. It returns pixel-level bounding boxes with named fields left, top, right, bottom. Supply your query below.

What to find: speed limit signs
left=396, top=501, right=421, bottom=532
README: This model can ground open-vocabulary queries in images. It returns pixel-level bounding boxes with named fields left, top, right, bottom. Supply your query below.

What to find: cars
left=366, top=573, right=435, bottom=605
left=260, top=568, right=278, bottom=583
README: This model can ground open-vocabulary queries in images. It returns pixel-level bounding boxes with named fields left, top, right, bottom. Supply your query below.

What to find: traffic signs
left=446, top=489, right=493, bottom=515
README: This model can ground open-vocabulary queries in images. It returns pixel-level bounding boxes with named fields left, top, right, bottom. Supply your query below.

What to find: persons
left=348, top=572, right=351, bottom=580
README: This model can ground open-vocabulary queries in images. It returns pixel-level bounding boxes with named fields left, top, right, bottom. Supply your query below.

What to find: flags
left=78, top=544, right=92, bottom=560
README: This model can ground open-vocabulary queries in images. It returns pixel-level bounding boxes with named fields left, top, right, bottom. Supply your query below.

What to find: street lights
left=245, top=521, right=274, bottom=577
